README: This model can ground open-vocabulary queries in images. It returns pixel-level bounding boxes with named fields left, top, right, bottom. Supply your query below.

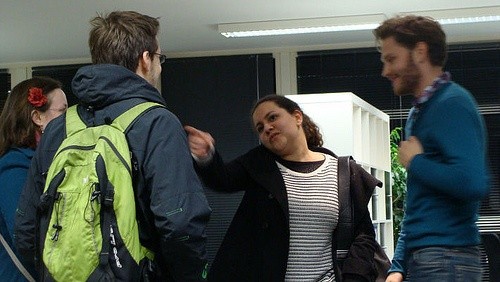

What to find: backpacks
left=34, top=102, right=166, bottom=282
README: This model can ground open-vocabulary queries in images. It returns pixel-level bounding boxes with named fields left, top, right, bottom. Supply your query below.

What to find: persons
left=373, top=14, right=491, bottom=282
left=185, top=94, right=382, bottom=282
left=12, top=10, right=214, bottom=282
left=0, top=77, right=69, bottom=282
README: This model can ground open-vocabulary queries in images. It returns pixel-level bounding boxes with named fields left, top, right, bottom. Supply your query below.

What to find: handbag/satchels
left=333, top=156, right=392, bottom=282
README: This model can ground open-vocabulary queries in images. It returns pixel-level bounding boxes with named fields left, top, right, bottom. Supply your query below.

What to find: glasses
left=141, top=48, right=166, bottom=64
left=40, top=107, right=66, bottom=114
